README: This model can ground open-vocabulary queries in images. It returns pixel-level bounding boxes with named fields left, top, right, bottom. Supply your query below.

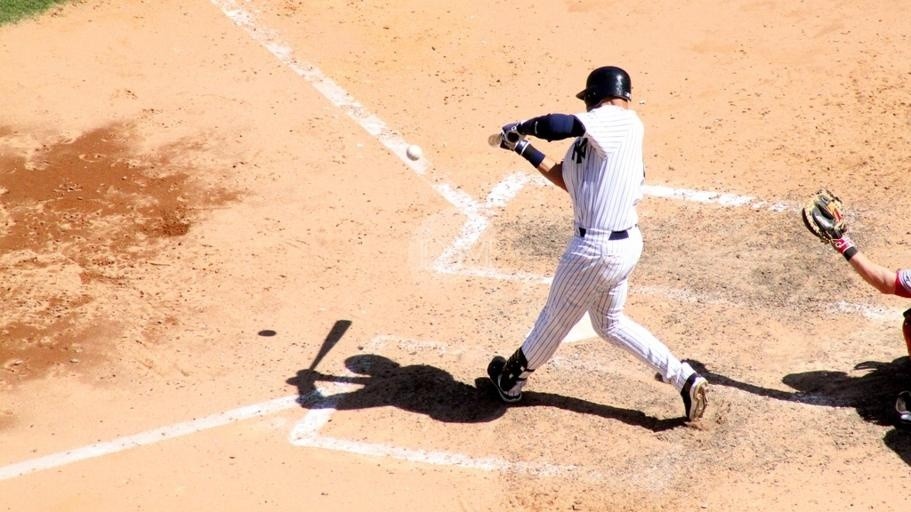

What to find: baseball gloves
left=802, top=187, right=848, bottom=245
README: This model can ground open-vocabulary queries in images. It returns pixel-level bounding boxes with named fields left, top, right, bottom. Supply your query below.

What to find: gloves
left=500, top=120, right=530, bottom=156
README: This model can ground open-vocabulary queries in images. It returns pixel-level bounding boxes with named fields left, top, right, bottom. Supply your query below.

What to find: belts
left=579, top=227, right=629, bottom=240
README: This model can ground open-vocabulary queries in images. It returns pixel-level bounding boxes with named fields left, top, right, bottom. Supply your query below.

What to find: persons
left=486, top=63, right=710, bottom=421
left=802, top=188, right=911, bottom=423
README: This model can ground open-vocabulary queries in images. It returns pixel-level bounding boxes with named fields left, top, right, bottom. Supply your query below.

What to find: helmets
left=576, top=66, right=631, bottom=112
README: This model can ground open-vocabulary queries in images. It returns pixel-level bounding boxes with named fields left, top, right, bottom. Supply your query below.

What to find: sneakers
left=896, top=391, right=911, bottom=422
left=488, top=356, right=522, bottom=402
left=681, top=373, right=709, bottom=419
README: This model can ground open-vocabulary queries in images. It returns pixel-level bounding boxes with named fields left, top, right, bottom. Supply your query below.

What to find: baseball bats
left=488, top=129, right=521, bottom=146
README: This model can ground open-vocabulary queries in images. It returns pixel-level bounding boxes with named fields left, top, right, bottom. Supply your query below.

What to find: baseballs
left=406, top=144, right=423, bottom=161
